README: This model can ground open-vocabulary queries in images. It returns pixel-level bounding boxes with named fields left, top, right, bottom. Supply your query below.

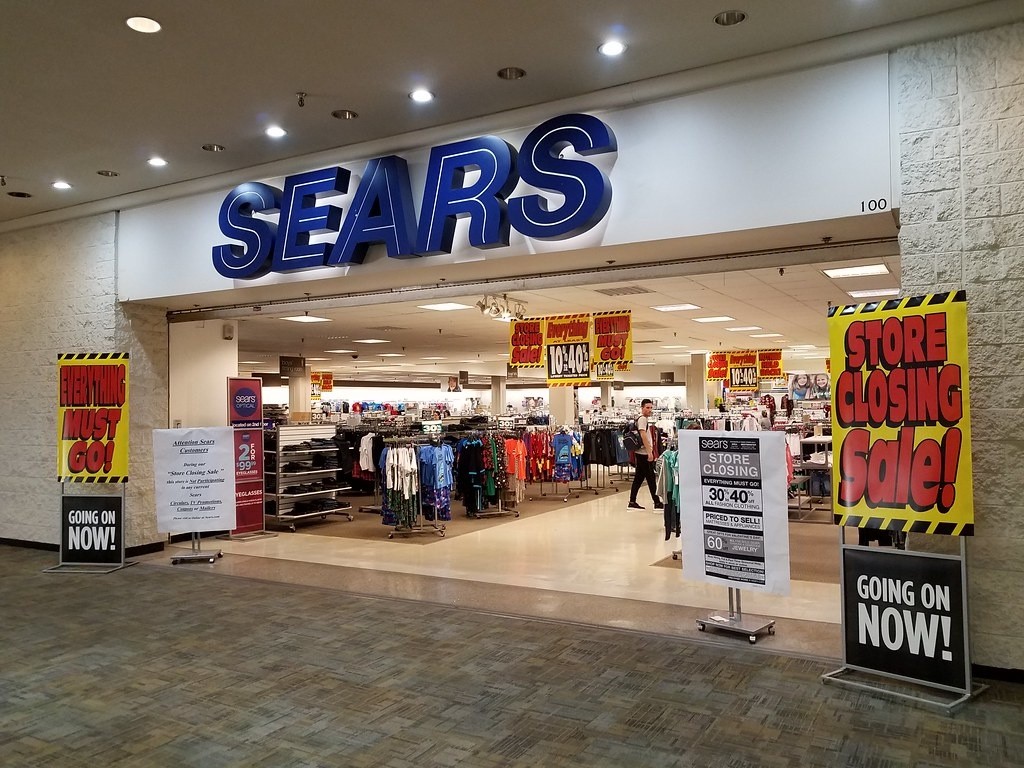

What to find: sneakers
left=653, top=502, right=664, bottom=510
left=627, top=502, right=645, bottom=510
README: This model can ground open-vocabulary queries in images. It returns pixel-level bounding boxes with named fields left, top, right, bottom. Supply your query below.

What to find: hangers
left=467, top=430, right=480, bottom=443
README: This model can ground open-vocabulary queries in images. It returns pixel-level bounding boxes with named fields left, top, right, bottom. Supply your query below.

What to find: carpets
left=390, top=438, right=414, bottom=450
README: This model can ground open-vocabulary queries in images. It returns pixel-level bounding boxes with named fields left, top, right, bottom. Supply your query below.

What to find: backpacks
left=622, top=415, right=648, bottom=450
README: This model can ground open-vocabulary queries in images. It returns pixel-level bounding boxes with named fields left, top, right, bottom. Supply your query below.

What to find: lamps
left=476, top=292, right=528, bottom=321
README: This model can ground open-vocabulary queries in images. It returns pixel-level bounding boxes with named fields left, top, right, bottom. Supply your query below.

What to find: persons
left=789, top=374, right=813, bottom=399
left=759, top=395, right=775, bottom=425
left=781, top=393, right=794, bottom=418
left=444, top=410, right=450, bottom=418
left=626, top=398, right=664, bottom=510
left=433, top=411, right=441, bottom=420
left=810, top=374, right=831, bottom=398
left=447, top=376, right=462, bottom=392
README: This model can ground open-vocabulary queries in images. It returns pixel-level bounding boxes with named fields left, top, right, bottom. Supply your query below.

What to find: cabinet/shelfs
left=788, top=435, right=834, bottom=525
left=263, top=409, right=353, bottom=533
